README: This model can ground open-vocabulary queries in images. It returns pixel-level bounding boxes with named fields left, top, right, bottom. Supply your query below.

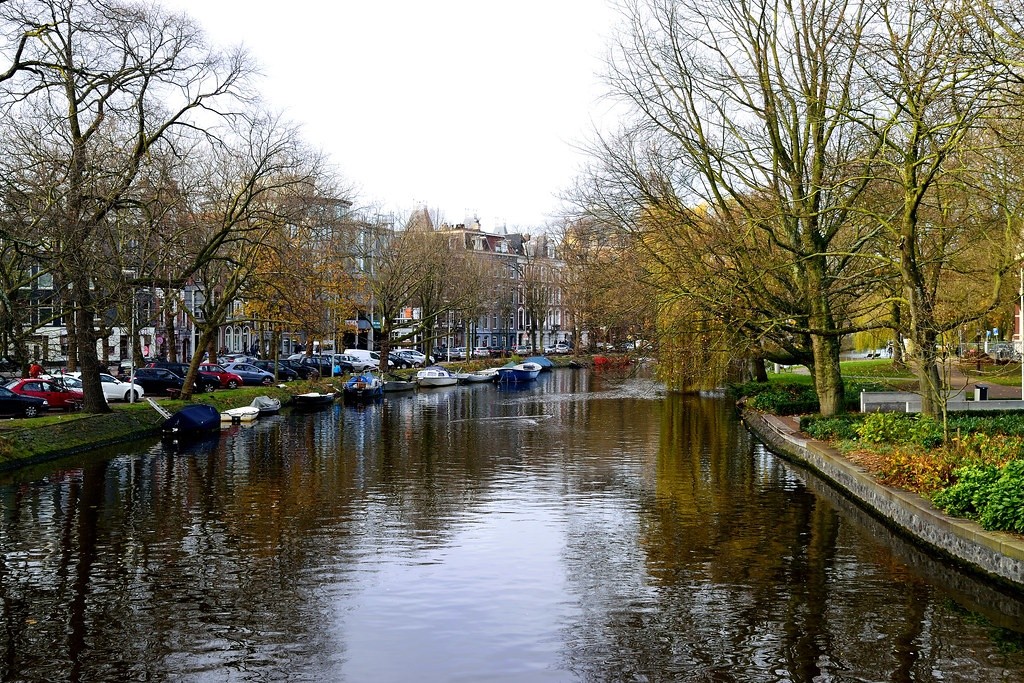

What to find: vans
left=299, top=341, right=336, bottom=354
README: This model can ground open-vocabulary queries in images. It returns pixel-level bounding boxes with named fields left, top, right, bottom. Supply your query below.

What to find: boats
left=158, top=403, right=221, bottom=437
left=250, top=396, right=282, bottom=413
left=455, top=361, right=542, bottom=382
left=341, top=371, right=383, bottom=397
left=594, top=355, right=655, bottom=365
left=290, top=392, right=335, bottom=402
left=414, top=367, right=459, bottom=386
left=220, top=406, right=260, bottom=422
left=381, top=380, right=417, bottom=391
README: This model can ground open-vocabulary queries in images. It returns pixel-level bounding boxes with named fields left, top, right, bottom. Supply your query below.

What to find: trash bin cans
left=974, top=384, right=988, bottom=401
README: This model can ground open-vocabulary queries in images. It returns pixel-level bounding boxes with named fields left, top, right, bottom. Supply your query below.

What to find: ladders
left=147, top=397, right=173, bottom=419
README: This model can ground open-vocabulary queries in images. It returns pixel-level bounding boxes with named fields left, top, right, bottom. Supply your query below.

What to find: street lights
left=122, top=270, right=136, bottom=401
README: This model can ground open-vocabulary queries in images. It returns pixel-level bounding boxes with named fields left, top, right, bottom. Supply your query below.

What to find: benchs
left=905, top=399, right=1024, bottom=413
left=859, top=390, right=967, bottom=414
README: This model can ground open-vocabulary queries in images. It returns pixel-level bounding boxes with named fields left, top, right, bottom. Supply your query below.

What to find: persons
left=294, top=343, right=319, bottom=354
left=99, top=353, right=168, bottom=376
left=186, top=344, right=262, bottom=363
left=23, top=360, right=44, bottom=380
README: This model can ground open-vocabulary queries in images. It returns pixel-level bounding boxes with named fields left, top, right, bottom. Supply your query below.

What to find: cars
left=0, top=372, right=145, bottom=418
left=433, top=344, right=569, bottom=360
left=123, top=352, right=320, bottom=394
left=290, top=349, right=437, bottom=379
left=988, top=343, right=1012, bottom=355
left=956, top=344, right=976, bottom=357
